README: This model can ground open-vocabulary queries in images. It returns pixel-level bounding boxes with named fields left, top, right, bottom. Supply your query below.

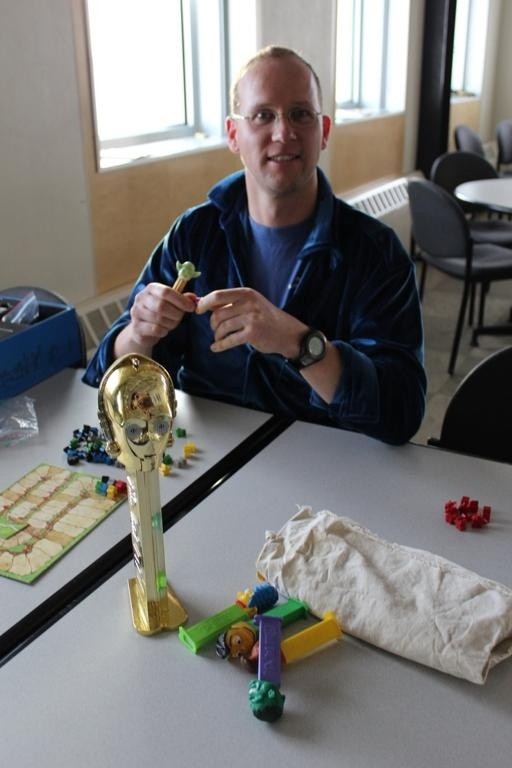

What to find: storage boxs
left=0, top=296, right=82, bottom=401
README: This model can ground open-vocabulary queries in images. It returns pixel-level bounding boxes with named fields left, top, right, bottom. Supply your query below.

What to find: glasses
left=229, top=107, right=321, bottom=124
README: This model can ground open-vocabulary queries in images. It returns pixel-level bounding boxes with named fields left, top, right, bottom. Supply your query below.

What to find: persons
left=80, top=45, right=427, bottom=446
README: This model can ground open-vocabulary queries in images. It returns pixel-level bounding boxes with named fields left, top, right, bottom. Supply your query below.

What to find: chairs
left=487, top=120, right=512, bottom=220
left=429, top=151, right=512, bottom=327
left=426, top=346, right=512, bottom=464
left=455, top=124, right=486, bottom=158
left=0, top=286, right=87, bottom=368
left=405, top=176, right=511, bottom=374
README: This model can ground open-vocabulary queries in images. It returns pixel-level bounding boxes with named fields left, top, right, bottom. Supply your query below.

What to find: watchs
left=283, top=327, right=328, bottom=371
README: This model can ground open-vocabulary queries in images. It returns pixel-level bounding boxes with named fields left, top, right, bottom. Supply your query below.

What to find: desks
left=454, top=177, right=512, bottom=214
left=1, top=368, right=512, bottom=767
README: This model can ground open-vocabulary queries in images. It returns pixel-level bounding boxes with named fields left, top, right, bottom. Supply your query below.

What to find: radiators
left=79, top=170, right=421, bottom=355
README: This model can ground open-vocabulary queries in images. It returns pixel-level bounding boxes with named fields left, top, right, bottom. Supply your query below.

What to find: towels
left=256, top=505, right=512, bottom=687
